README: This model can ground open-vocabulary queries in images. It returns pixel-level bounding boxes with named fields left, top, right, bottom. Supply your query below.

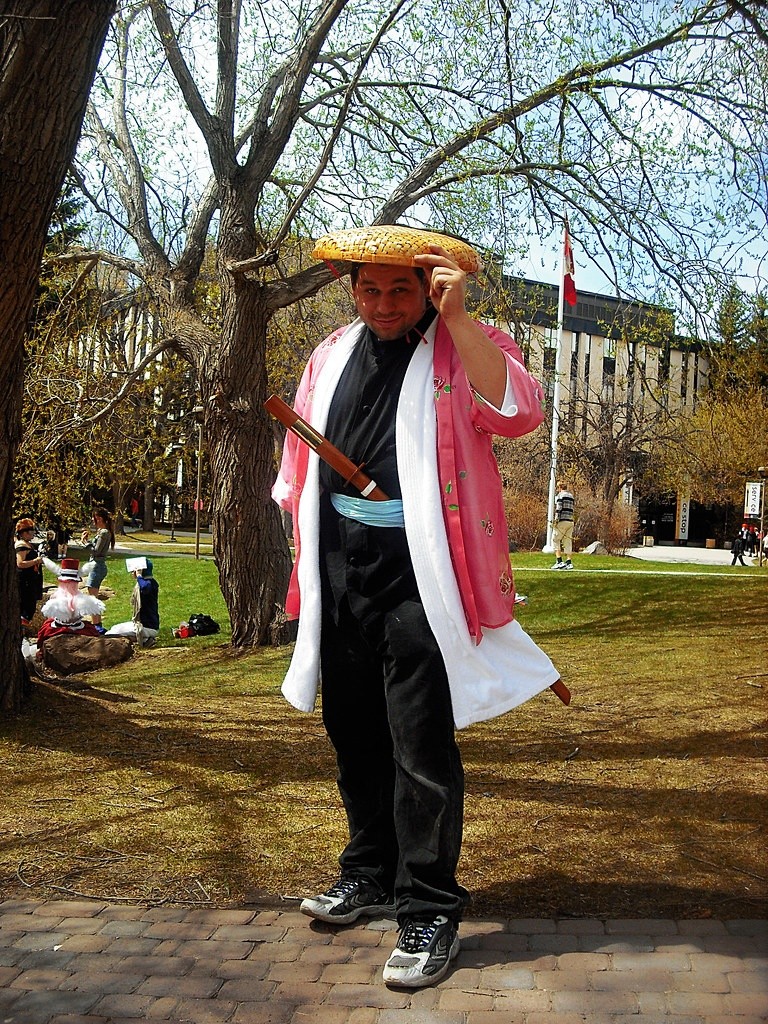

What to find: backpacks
left=188, top=614, right=220, bottom=636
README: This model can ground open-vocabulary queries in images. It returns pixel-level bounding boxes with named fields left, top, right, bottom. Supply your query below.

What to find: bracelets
left=33, top=558, right=38, bottom=564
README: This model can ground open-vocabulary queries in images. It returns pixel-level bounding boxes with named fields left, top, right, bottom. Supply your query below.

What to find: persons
left=551, top=482, right=576, bottom=570
left=15, top=498, right=161, bottom=646
left=270, top=223, right=544, bottom=989
left=715, top=523, right=768, bottom=566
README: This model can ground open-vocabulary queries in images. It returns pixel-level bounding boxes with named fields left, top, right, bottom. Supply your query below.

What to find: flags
left=563, top=224, right=577, bottom=307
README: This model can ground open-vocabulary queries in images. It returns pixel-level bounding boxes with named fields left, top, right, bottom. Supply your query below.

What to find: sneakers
left=300, top=870, right=397, bottom=924
left=551, top=561, right=565, bottom=569
left=561, top=564, right=573, bottom=570
left=382, top=913, right=460, bottom=987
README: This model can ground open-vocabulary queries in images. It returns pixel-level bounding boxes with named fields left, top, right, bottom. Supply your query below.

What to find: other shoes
left=93, top=623, right=108, bottom=635
left=143, top=638, right=158, bottom=648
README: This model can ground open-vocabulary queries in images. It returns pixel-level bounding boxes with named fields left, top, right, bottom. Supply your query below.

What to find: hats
left=57, top=559, right=82, bottom=582
left=311, top=225, right=479, bottom=273
